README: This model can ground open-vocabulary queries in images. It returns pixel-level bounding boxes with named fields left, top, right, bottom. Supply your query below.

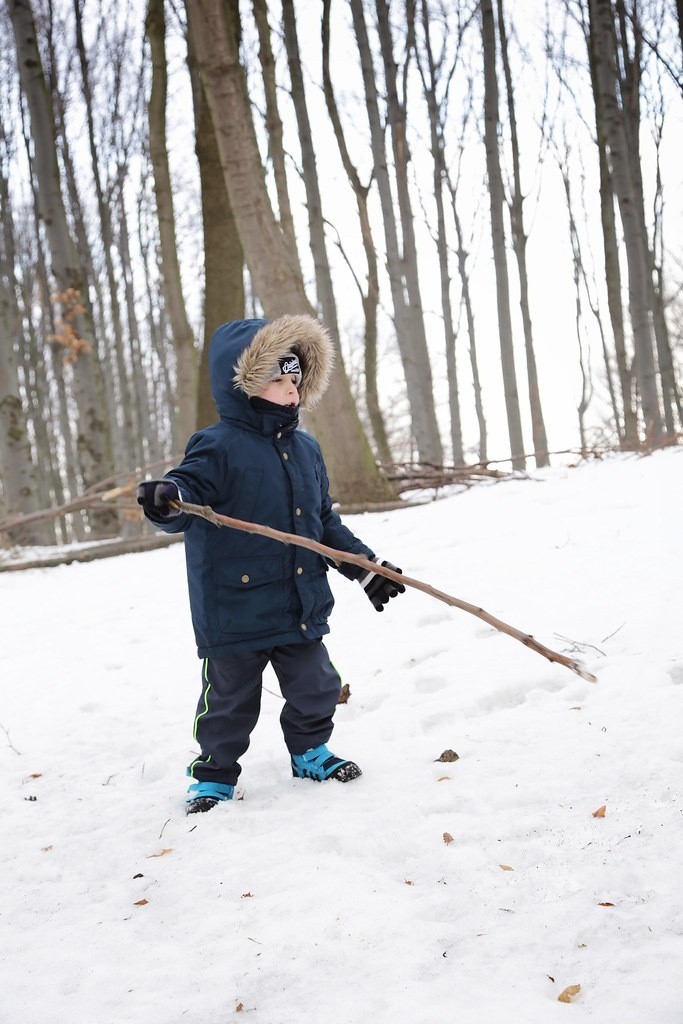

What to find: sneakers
left=186, top=781, right=233, bottom=815
left=291, top=744, right=362, bottom=783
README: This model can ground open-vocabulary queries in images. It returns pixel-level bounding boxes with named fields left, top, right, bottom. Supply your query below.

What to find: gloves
left=137, top=481, right=180, bottom=517
left=355, top=556, right=405, bottom=612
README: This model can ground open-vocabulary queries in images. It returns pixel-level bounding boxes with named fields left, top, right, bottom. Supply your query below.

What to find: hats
left=269, top=349, right=302, bottom=387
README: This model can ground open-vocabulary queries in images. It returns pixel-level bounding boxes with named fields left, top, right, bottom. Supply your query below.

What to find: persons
left=137, top=313, right=405, bottom=812
